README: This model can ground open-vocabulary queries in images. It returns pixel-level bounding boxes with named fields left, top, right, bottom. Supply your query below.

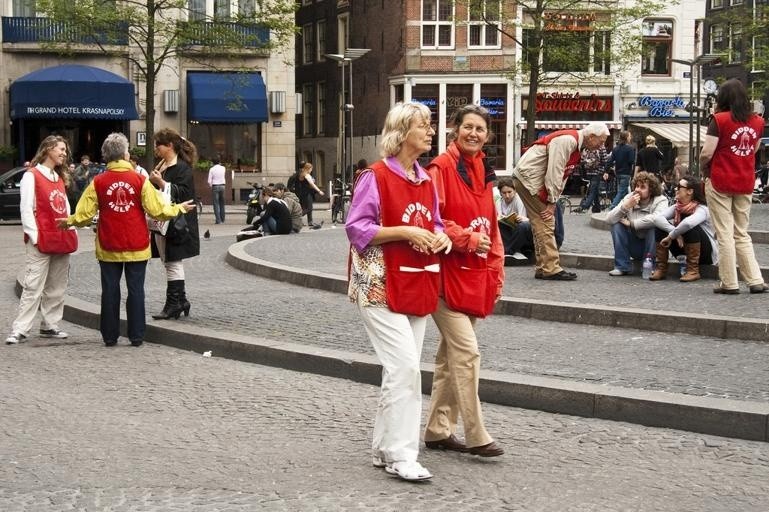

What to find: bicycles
left=557, top=194, right=574, bottom=215
left=329, top=179, right=355, bottom=225
left=195, top=194, right=203, bottom=222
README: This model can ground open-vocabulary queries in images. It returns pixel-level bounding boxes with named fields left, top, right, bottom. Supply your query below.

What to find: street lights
left=323, top=44, right=374, bottom=197
left=668, top=53, right=724, bottom=177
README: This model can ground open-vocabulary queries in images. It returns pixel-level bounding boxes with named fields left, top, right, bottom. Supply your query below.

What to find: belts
left=211, top=184, right=225, bottom=186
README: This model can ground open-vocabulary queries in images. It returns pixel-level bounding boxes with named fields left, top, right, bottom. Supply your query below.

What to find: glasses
left=415, top=119, right=439, bottom=133
left=674, top=181, right=690, bottom=192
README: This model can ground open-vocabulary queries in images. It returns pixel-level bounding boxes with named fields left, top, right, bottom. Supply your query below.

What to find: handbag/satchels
left=167, top=184, right=190, bottom=247
left=143, top=182, right=171, bottom=236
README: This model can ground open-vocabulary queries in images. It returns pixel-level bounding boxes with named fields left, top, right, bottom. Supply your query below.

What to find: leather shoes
left=467, top=441, right=504, bottom=458
left=542, top=270, right=577, bottom=282
left=424, top=433, right=470, bottom=456
left=533, top=272, right=542, bottom=280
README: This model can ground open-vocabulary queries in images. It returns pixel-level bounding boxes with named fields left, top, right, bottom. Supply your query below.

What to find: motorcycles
left=244, top=180, right=275, bottom=225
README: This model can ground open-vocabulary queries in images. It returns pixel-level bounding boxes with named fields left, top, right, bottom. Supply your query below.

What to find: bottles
left=680, top=255, right=686, bottom=277
left=642, top=258, right=653, bottom=279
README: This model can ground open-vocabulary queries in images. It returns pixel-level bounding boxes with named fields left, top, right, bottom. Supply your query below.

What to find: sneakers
left=371, top=455, right=385, bottom=469
left=749, top=282, right=769, bottom=294
left=712, top=285, right=740, bottom=295
left=608, top=269, right=626, bottom=276
left=383, top=459, right=433, bottom=481
left=5, top=332, right=26, bottom=344
left=38, top=328, right=67, bottom=339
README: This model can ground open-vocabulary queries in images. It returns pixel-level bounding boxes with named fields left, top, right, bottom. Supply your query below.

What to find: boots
left=150, top=279, right=181, bottom=321
left=679, top=242, right=700, bottom=282
left=177, top=280, right=189, bottom=317
left=648, top=241, right=669, bottom=281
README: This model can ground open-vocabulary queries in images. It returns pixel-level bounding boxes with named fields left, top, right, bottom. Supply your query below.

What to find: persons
left=146, top=127, right=201, bottom=323
left=206, top=158, right=235, bottom=226
left=53, top=130, right=197, bottom=349
left=699, top=80, right=769, bottom=295
left=572, top=130, right=719, bottom=280
left=510, top=123, right=610, bottom=279
left=750, top=145, right=769, bottom=204
left=241, top=158, right=369, bottom=237
left=342, top=98, right=455, bottom=482
left=23, top=154, right=151, bottom=189
left=5, top=134, right=81, bottom=346
left=422, top=103, right=509, bottom=459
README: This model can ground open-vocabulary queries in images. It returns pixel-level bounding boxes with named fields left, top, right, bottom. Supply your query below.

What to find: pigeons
left=309, top=220, right=325, bottom=230
left=204, top=229, right=211, bottom=239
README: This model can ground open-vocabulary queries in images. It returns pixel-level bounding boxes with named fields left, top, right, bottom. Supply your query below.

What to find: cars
left=0, top=163, right=83, bottom=221
